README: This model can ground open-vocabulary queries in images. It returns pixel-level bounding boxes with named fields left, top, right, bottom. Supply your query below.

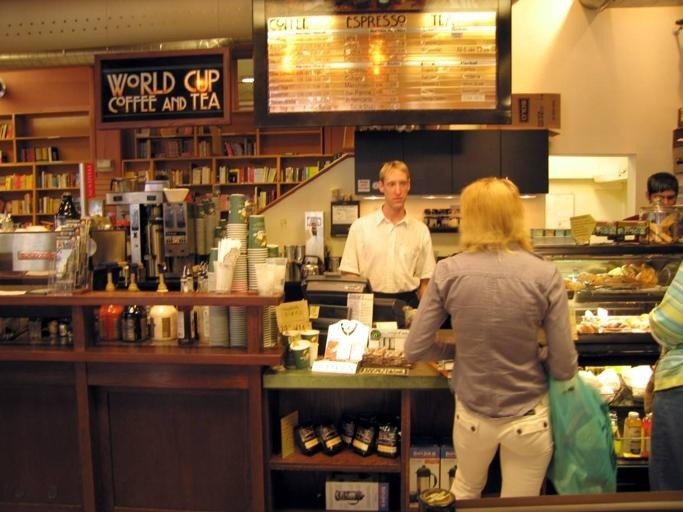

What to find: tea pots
left=296, top=255, right=327, bottom=278
left=110, top=177, right=128, bottom=192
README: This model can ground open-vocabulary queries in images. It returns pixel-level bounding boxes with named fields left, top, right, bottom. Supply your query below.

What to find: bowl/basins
left=163, top=188, right=190, bottom=202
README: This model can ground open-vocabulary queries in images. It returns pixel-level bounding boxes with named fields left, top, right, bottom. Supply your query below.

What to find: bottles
left=149, top=263, right=177, bottom=342
left=121, top=263, right=147, bottom=342
left=178, top=265, right=207, bottom=344
left=54, top=195, right=79, bottom=229
left=623, top=411, right=641, bottom=454
left=99, top=271, right=122, bottom=341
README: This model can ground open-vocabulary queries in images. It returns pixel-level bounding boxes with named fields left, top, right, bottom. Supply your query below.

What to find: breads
left=564, top=264, right=659, bottom=291
left=577, top=314, right=650, bottom=333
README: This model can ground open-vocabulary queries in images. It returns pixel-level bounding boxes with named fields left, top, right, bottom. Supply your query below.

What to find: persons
left=622, top=172, right=679, bottom=220
left=648, top=262, right=682, bottom=491
left=405, top=177, right=577, bottom=501
left=339, top=160, right=435, bottom=309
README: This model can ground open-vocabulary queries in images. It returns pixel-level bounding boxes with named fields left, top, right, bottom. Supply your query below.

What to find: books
left=223, top=138, right=257, bottom=155
left=286, top=161, right=329, bottom=182
left=0, top=194, right=30, bottom=213
left=157, top=168, right=186, bottom=186
left=138, top=127, right=192, bottom=135
left=251, top=185, right=276, bottom=213
left=36, top=196, right=61, bottom=213
left=198, top=128, right=206, bottom=133
left=197, top=141, right=210, bottom=155
left=19, top=145, right=61, bottom=162
left=0, top=172, right=32, bottom=186
left=139, top=138, right=192, bottom=159
left=37, top=171, right=78, bottom=186
left=218, top=166, right=277, bottom=184
left=126, top=171, right=150, bottom=181
left=0, top=124, right=13, bottom=138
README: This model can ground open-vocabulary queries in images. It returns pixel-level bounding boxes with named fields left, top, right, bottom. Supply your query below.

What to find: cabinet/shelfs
left=0, top=66, right=93, bottom=268
left=262, top=360, right=513, bottom=512
left=101, top=110, right=356, bottom=232
left=530, top=241, right=683, bottom=496
left=0, top=292, right=284, bottom=509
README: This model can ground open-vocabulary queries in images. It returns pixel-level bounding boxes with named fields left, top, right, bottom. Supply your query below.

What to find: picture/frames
left=94, top=48, right=230, bottom=131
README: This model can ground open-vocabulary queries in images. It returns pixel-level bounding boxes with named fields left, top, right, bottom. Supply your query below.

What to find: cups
left=208, top=306, right=228, bottom=346
left=270, top=306, right=279, bottom=345
left=290, top=340, right=311, bottom=370
left=216, top=226, right=223, bottom=237
left=300, top=330, right=320, bottom=343
left=205, top=201, right=217, bottom=253
left=230, top=306, right=248, bottom=346
left=247, top=215, right=268, bottom=292
left=226, top=194, right=247, bottom=294
left=293, top=264, right=303, bottom=281
left=265, top=257, right=288, bottom=293
left=195, top=204, right=205, bottom=255
left=220, top=219, right=227, bottom=230
left=284, top=245, right=295, bottom=262
left=267, top=244, right=279, bottom=258
left=285, top=263, right=293, bottom=281
left=296, top=245, right=305, bottom=263
left=285, top=348, right=297, bottom=369
left=255, top=263, right=276, bottom=296
left=207, top=248, right=218, bottom=291
left=282, top=330, right=299, bottom=349
left=263, top=306, right=270, bottom=347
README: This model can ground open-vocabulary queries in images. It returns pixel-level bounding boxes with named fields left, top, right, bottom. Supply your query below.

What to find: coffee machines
left=105, top=192, right=196, bottom=291
